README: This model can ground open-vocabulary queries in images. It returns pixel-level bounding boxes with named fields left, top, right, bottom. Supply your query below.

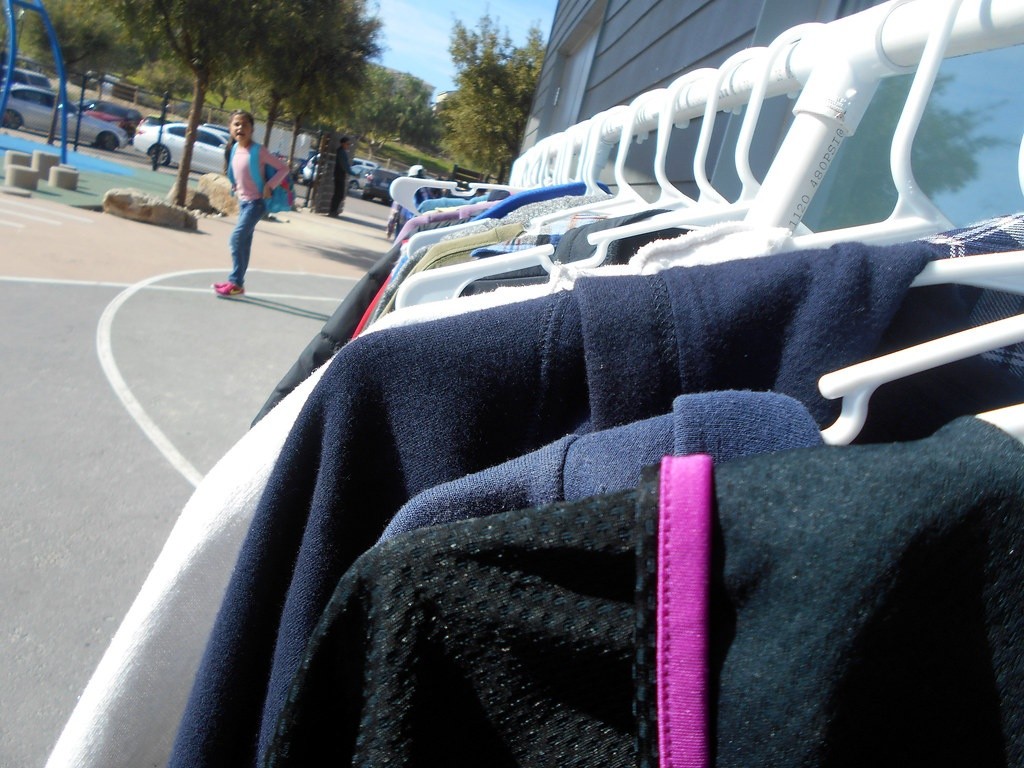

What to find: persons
left=305, top=147, right=322, bottom=207
left=328, top=136, right=359, bottom=218
left=387, top=163, right=428, bottom=238
left=211, top=110, right=291, bottom=299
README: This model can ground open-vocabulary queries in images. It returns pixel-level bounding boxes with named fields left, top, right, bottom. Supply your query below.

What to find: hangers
left=390, top=0, right=1024, bottom=446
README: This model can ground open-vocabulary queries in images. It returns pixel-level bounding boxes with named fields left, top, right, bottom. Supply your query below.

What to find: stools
left=48, top=164, right=79, bottom=192
left=5, top=164, right=40, bottom=192
left=4, top=150, right=32, bottom=167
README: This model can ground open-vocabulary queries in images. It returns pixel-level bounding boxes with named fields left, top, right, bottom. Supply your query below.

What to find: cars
left=133, top=122, right=230, bottom=178
left=303, top=153, right=320, bottom=185
left=348, top=165, right=376, bottom=189
left=0, top=84, right=128, bottom=152
left=136, top=114, right=230, bottom=140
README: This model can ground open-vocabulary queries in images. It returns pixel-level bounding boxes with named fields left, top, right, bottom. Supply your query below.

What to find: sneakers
left=214, top=284, right=246, bottom=297
left=211, top=282, right=228, bottom=288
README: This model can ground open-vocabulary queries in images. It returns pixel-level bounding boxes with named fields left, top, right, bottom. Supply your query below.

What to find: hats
left=408, top=165, right=424, bottom=176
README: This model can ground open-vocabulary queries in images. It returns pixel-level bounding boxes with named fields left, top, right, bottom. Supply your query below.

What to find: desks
left=31, top=150, right=59, bottom=180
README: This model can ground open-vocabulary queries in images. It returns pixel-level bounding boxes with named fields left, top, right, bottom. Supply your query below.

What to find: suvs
left=0, top=64, right=50, bottom=91
left=70, top=98, right=141, bottom=137
left=361, top=168, right=402, bottom=206
